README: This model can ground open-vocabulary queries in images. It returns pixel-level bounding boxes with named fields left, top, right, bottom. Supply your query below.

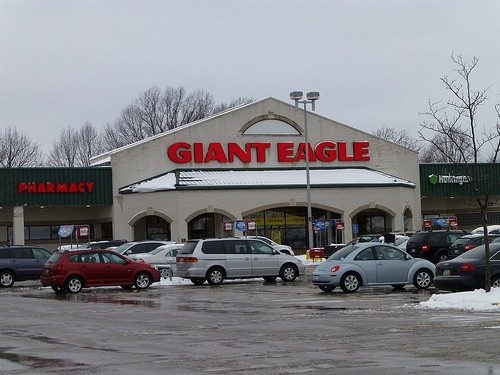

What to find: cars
left=240, top=236, right=295, bottom=256
left=57, top=238, right=186, bottom=280
left=311, top=242, right=436, bottom=293
left=173, top=236, right=305, bottom=286
left=345, top=224, right=500, bottom=292
left=0, top=243, right=54, bottom=288
left=39, top=249, right=161, bottom=295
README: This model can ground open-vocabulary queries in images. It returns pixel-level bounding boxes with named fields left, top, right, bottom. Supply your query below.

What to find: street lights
left=289, top=90, right=320, bottom=251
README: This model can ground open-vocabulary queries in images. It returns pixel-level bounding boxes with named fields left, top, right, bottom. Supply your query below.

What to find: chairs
left=84, top=256, right=96, bottom=263
left=240, top=247, right=245, bottom=253
left=380, top=249, right=391, bottom=260
